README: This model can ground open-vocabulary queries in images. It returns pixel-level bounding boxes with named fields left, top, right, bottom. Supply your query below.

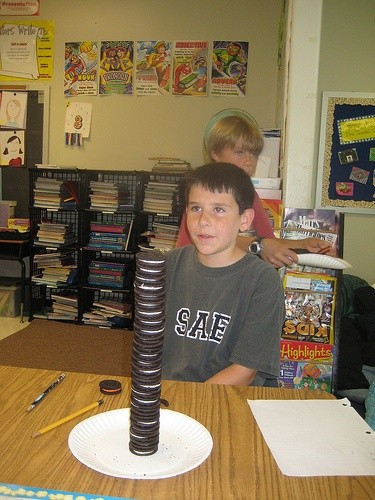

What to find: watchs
left=248, top=237, right=266, bottom=260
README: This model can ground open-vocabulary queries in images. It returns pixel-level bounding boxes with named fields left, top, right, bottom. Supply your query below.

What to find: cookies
left=99, top=380, right=122, bottom=395
left=129, top=250, right=165, bottom=455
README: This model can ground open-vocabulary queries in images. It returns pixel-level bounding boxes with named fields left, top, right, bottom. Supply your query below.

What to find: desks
left=0, top=240, right=29, bottom=323
left=0, top=365, right=375, bottom=500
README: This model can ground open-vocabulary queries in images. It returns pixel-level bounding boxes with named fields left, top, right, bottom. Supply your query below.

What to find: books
left=88, top=258, right=133, bottom=288
left=35, top=157, right=193, bottom=173
left=143, top=180, right=182, bottom=213
left=33, top=217, right=77, bottom=247
left=81, top=298, right=134, bottom=327
left=33, top=176, right=77, bottom=209
left=30, top=250, right=78, bottom=287
left=86, top=220, right=130, bottom=251
left=88, top=180, right=130, bottom=211
left=138, top=221, right=181, bottom=252
left=32, top=290, right=79, bottom=321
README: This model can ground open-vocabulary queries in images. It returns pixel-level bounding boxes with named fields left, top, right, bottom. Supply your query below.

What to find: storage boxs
left=0, top=285, right=22, bottom=318
left=254, top=137, right=281, bottom=177
left=0, top=200, right=17, bottom=218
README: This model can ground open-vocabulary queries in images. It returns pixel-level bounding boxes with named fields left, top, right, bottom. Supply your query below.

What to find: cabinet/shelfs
left=27, top=167, right=186, bottom=330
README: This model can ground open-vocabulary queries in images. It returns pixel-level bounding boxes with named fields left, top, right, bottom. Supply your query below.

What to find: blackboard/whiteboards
left=0, top=83, right=49, bottom=223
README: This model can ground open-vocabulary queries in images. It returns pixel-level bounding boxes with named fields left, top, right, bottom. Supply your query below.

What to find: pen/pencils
left=25, top=372, right=67, bottom=414
left=30, top=398, right=105, bottom=439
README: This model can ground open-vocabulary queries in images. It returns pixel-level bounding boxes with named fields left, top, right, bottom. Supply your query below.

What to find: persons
left=160, top=162, right=287, bottom=388
left=173, top=116, right=336, bottom=269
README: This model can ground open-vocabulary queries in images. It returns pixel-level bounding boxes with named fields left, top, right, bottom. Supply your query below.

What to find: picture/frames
left=314, top=92, right=375, bottom=215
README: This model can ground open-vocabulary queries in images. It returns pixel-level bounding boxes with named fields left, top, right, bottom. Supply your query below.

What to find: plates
left=287, top=254, right=352, bottom=269
left=68, top=408, right=213, bottom=479
